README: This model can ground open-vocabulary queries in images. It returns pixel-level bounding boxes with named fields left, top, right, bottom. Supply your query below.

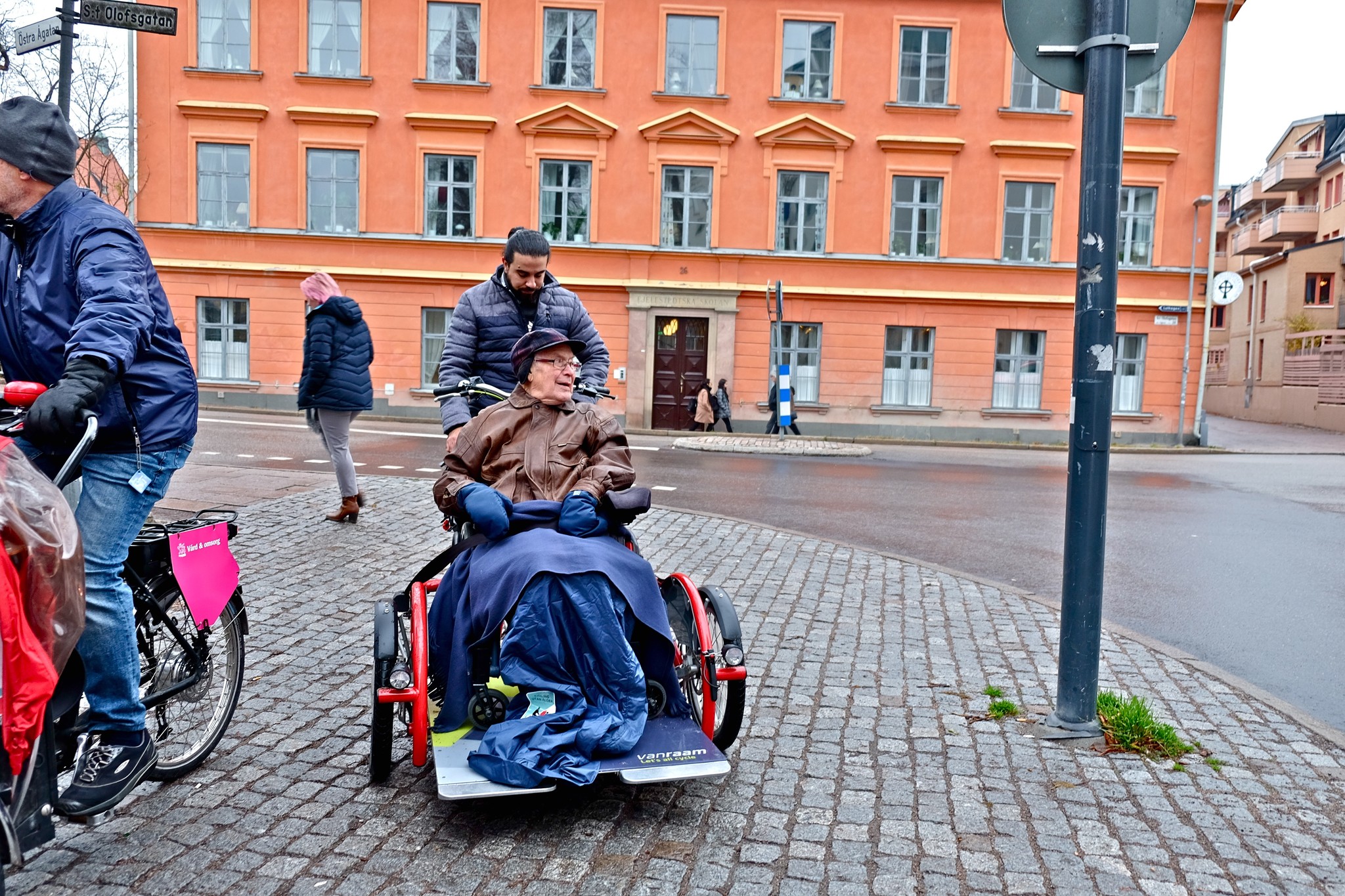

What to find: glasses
left=534, top=358, right=582, bottom=372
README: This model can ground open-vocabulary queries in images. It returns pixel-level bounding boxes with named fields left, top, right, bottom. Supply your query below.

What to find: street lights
left=1176, top=194, right=1214, bottom=448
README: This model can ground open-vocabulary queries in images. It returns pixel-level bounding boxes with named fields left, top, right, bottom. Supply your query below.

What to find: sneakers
left=56, top=725, right=158, bottom=817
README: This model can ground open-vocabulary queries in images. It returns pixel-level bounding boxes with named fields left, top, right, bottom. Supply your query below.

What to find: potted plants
left=427, top=200, right=444, bottom=236
left=1282, top=310, right=1325, bottom=355
left=891, top=236, right=925, bottom=257
left=569, top=209, right=586, bottom=243
left=542, top=221, right=560, bottom=240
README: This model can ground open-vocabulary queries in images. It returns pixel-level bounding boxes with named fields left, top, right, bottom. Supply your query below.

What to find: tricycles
left=369, top=375, right=749, bottom=804
left=0, top=377, right=248, bottom=895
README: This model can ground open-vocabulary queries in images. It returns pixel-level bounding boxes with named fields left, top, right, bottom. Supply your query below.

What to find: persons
left=439, top=226, right=610, bottom=451
left=0, top=96, right=198, bottom=818
left=296, top=271, right=374, bottom=524
left=432, top=327, right=635, bottom=707
left=766, top=367, right=801, bottom=435
left=686, top=379, right=733, bottom=433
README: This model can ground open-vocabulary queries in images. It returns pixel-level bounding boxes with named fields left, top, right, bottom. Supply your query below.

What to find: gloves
left=23, top=360, right=114, bottom=447
left=457, top=483, right=514, bottom=540
left=558, top=490, right=608, bottom=539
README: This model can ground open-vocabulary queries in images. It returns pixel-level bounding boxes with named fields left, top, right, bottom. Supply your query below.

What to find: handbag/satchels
left=711, top=395, right=720, bottom=413
left=687, top=398, right=697, bottom=414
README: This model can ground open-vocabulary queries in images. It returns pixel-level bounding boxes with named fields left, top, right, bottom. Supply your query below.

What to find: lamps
left=1032, top=242, right=1044, bottom=262
left=925, top=237, right=936, bottom=256
left=669, top=72, right=681, bottom=92
left=448, top=66, right=462, bottom=81
left=563, top=69, right=578, bottom=86
left=222, top=53, right=232, bottom=69
left=236, top=202, right=248, bottom=227
left=330, top=59, right=340, bottom=75
left=812, top=80, right=823, bottom=97
left=455, top=224, right=465, bottom=236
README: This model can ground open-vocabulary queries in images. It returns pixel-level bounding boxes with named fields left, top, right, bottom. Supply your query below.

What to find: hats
left=0, top=95, right=80, bottom=186
left=510, top=327, right=588, bottom=376
left=770, top=369, right=776, bottom=377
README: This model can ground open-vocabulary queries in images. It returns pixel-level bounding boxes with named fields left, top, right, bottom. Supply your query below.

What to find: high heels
left=326, top=496, right=359, bottom=523
left=355, top=484, right=365, bottom=508
